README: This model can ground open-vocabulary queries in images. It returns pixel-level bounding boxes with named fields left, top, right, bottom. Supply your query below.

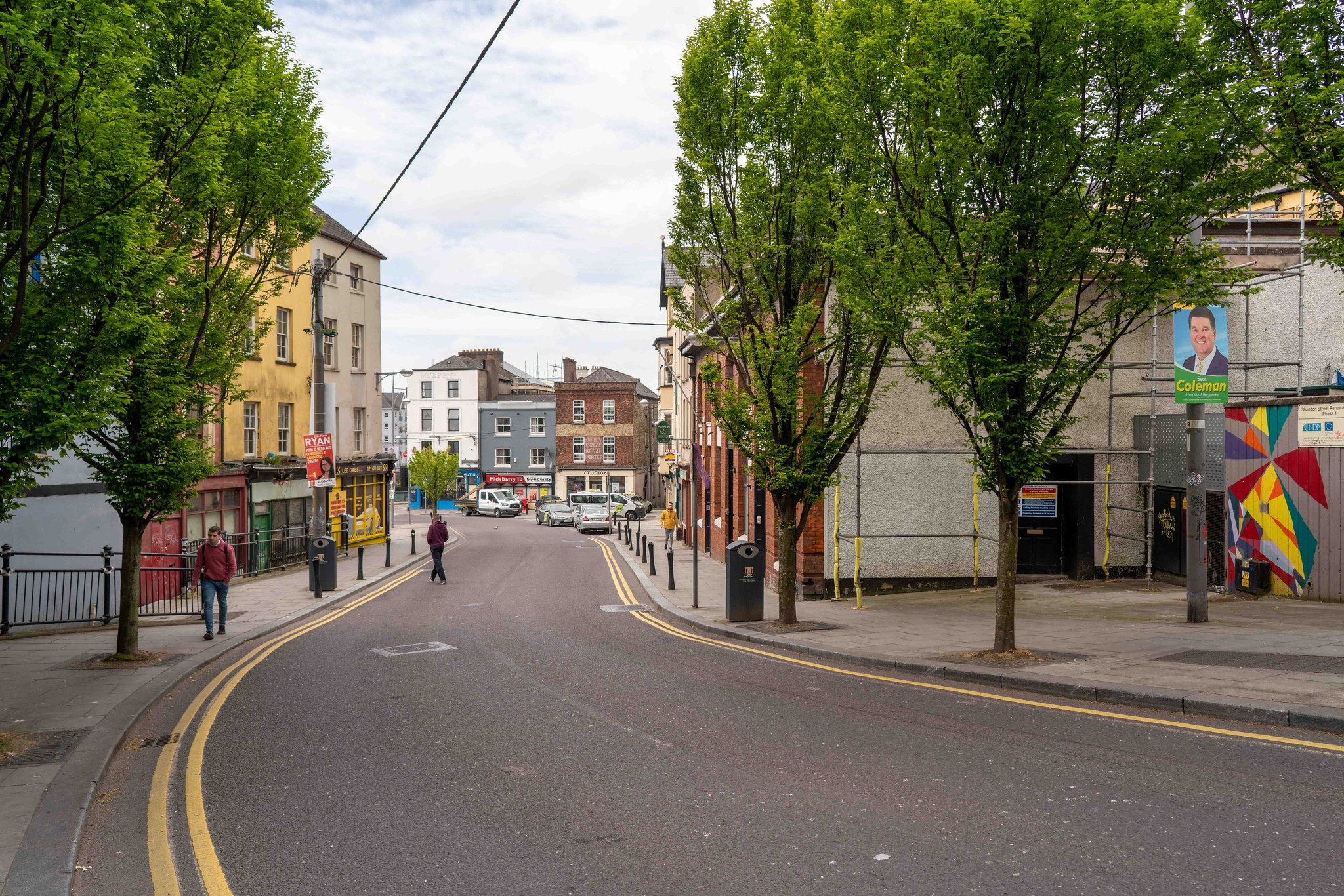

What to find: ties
left=1197, top=362, right=1203, bottom=373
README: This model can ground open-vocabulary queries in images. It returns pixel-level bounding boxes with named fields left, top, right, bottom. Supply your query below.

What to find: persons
left=589, top=495, right=594, bottom=502
left=660, top=502, right=678, bottom=551
left=309, top=479, right=317, bottom=488
left=316, top=457, right=334, bottom=481
left=389, top=448, right=394, bottom=454
left=532, top=494, right=536, bottom=501
left=574, top=484, right=581, bottom=493
left=1182, top=306, right=1228, bottom=375
left=190, top=526, right=237, bottom=640
left=517, top=494, right=525, bottom=514
left=426, top=513, right=449, bottom=585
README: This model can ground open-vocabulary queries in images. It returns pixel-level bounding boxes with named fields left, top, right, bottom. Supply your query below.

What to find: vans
left=566, top=489, right=647, bottom=521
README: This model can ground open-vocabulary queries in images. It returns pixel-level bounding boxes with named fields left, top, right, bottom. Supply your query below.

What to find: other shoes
left=665, top=543, right=668, bottom=549
left=669, top=547, right=673, bottom=551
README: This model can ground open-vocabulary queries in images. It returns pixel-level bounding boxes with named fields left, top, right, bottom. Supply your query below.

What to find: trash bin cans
left=725, top=540, right=765, bottom=622
left=309, top=534, right=337, bottom=592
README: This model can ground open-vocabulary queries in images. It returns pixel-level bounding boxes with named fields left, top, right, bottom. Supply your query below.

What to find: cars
left=535, top=501, right=575, bottom=527
left=616, top=493, right=652, bottom=513
left=571, top=504, right=613, bottom=534
left=534, top=495, right=563, bottom=512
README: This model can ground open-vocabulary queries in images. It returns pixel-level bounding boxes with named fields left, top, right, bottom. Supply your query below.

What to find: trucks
left=453, top=487, right=522, bottom=518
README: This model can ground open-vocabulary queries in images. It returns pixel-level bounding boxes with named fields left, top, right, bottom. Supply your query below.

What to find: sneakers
left=217, top=625, right=226, bottom=634
left=429, top=575, right=434, bottom=582
left=204, top=631, right=214, bottom=640
left=437, top=580, right=447, bottom=584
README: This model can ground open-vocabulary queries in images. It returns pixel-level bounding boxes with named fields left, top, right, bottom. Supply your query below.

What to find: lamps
left=544, top=412, right=548, bottom=415
left=640, top=400, right=658, bottom=407
left=650, top=463, right=660, bottom=467
left=374, top=368, right=414, bottom=392
left=516, top=412, right=520, bottom=415
left=490, top=413, right=493, bottom=415
left=429, top=433, right=440, bottom=444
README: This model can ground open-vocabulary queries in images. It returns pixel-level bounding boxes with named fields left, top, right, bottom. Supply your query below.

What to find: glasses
left=322, top=463, right=330, bottom=466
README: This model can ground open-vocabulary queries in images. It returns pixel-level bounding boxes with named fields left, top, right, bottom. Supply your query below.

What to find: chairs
left=600, top=510, right=604, bottom=514
left=582, top=496, right=593, bottom=503
left=587, top=511, right=593, bottom=514
